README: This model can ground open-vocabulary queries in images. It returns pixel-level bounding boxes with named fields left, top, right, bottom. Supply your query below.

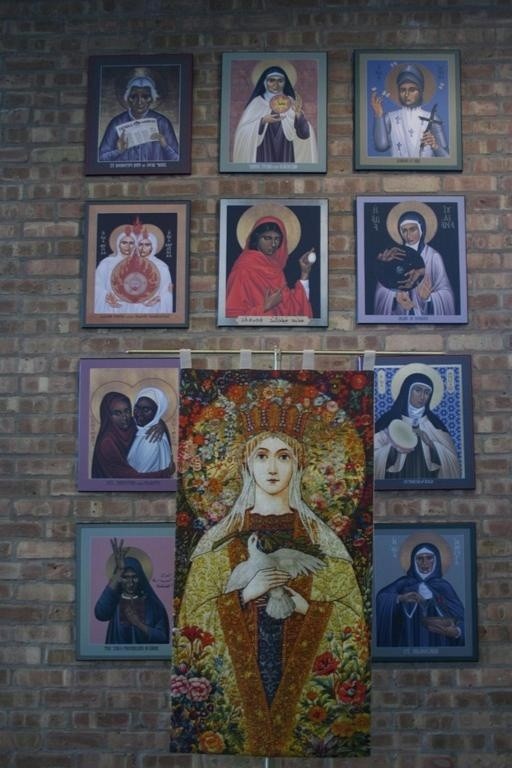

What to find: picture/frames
left=82, top=56, right=192, bottom=173
left=74, top=524, right=176, bottom=663
left=355, top=193, right=468, bottom=325
left=374, top=523, right=478, bottom=662
left=221, top=53, right=327, bottom=175
left=369, top=353, right=476, bottom=490
left=219, top=196, right=328, bottom=327
left=354, top=48, right=463, bottom=172
left=80, top=197, right=189, bottom=329
left=79, top=356, right=183, bottom=490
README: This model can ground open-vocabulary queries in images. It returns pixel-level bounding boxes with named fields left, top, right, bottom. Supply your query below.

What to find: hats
left=239, top=403, right=310, bottom=438
